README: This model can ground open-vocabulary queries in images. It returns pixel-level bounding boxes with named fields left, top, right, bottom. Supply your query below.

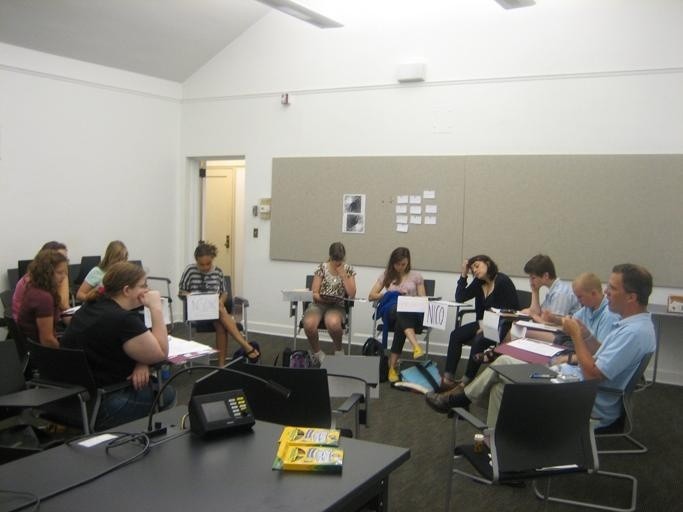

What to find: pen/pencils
left=529, top=374, right=557, bottom=378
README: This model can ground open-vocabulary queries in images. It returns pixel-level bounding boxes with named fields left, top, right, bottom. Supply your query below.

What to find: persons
left=176, top=240, right=261, bottom=368
left=301, top=242, right=358, bottom=364
left=10, top=239, right=179, bottom=438
left=542, top=271, right=622, bottom=366
left=472, top=252, right=581, bottom=365
left=367, top=246, right=428, bottom=383
left=438, top=254, right=518, bottom=394
left=427, top=264, right=658, bottom=434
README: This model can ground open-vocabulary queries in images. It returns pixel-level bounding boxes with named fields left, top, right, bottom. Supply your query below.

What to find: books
left=165, top=334, right=219, bottom=365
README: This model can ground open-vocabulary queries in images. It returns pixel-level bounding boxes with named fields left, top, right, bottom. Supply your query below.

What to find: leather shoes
left=442, top=372, right=457, bottom=389
left=425, top=387, right=469, bottom=413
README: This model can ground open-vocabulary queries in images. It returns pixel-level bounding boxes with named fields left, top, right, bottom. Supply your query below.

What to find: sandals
left=473, top=345, right=500, bottom=364
left=247, top=347, right=260, bottom=360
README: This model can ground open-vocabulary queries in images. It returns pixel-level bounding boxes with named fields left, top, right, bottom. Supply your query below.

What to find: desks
left=1, top=402, right=411, bottom=511
left=646, top=303, right=683, bottom=389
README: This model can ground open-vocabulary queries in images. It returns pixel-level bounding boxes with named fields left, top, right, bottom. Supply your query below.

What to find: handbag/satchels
left=233, top=341, right=259, bottom=363
left=362, top=338, right=388, bottom=382
left=401, top=361, right=441, bottom=391
left=289, top=350, right=312, bottom=368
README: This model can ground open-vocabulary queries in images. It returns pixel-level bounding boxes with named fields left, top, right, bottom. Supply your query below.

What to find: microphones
left=141, top=366, right=293, bottom=438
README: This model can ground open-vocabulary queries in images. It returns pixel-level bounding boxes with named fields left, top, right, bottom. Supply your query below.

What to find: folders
left=493, top=337, right=569, bottom=366
left=319, top=294, right=368, bottom=302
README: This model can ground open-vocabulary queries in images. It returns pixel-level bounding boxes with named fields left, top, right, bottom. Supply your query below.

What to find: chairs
left=26, top=334, right=178, bottom=434
left=182, top=273, right=250, bottom=374
left=0, top=254, right=103, bottom=350
left=128, top=256, right=175, bottom=336
left=246, top=363, right=366, bottom=439
left=0, top=339, right=91, bottom=467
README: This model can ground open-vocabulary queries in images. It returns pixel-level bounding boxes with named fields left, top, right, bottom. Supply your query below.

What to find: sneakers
left=388, top=369, right=398, bottom=382
left=315, top=350, right=326, bottom=363
left=414, top=346, right=424, bottom=358
